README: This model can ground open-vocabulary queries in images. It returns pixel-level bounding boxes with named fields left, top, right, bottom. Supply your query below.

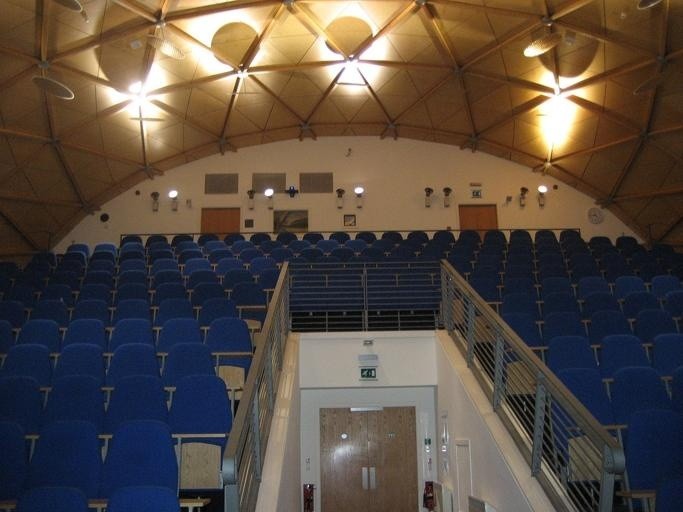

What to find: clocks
left=587, top=207, right=605, bottom=225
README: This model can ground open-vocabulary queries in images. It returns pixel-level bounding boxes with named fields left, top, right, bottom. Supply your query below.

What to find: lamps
left=246, top=190, right=255, bottom=209
left=519, top=187, right=528, bottom=206
left=264, top=188, right=274, bottom=209
left=354, top=187, right=363, bottom=208
left=168, top=190, right=177, bottom=210
left=537, top=185, right=547, bottom=206
left=443, top=188, right=451, bottom=207
left=425, top=188, right=433, bottom=207
left=336, top=189, right=345, bottom=209
left=150, top=191, right=160, bottom=212
